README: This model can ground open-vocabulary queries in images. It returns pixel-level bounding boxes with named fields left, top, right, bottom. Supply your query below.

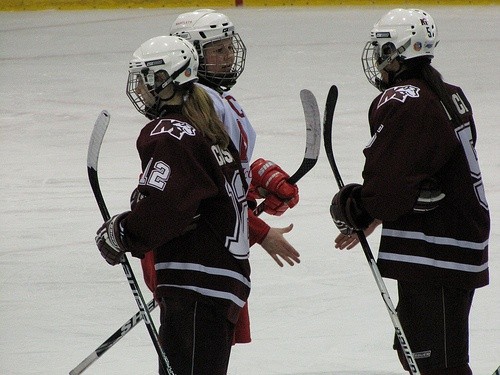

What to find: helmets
left=126, top=36, right=199, bottom=120
left=361, top=7, right=440, bottom=92
left=169, top=8, right=249, bottom=95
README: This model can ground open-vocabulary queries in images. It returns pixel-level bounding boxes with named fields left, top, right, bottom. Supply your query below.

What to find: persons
left=95, top=8, right=300, bottom=375
left=329, top=8, right=492, bottom=375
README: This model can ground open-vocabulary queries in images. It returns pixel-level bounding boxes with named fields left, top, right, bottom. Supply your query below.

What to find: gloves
left=249, top=158, right=299, bottom=217
left=94, top=211, right=134, bottom=266
left=329, top=183, right=374, bottom=237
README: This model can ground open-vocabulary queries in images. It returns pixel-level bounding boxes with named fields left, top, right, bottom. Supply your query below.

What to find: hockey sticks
left=84, top=107, right=175, bottom=375
left=60, top=89, right=321, bottom=375
left=322, top=84, right=421, bottom=375
